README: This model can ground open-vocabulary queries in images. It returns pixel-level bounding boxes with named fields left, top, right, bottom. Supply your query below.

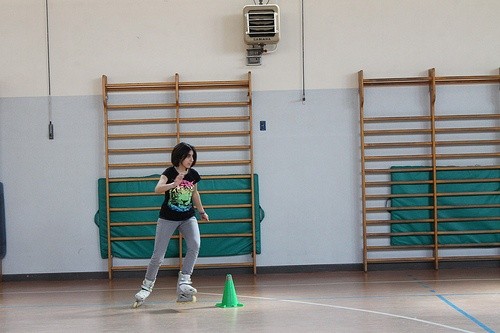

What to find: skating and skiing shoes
left=177, top=270, right=197, bottom=303
left=133, top=279, right=156, bottom=308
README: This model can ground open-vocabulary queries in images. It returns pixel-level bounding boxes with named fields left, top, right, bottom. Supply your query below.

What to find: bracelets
left=199, top=211, right=205, bottom=216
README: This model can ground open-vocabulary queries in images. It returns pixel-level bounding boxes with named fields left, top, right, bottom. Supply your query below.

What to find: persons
left=134, top=142, right=210, bottom=308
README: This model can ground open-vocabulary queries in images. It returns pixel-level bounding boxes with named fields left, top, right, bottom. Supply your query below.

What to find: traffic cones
left=215, top=274, right=244, bottom=308
